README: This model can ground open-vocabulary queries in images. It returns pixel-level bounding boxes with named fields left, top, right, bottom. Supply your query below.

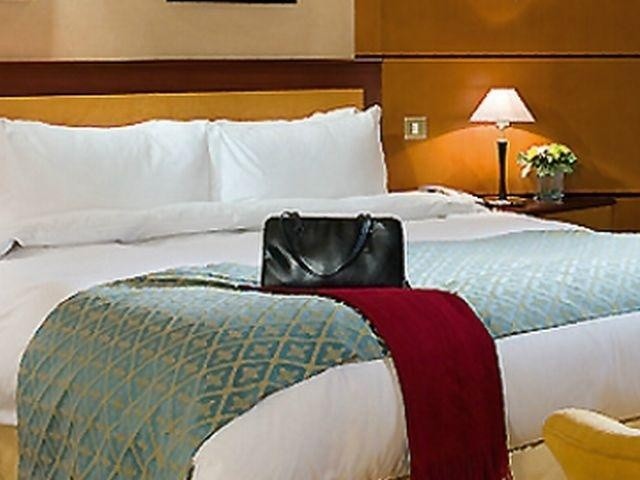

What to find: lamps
left=469, top=84, right=536, bottom=205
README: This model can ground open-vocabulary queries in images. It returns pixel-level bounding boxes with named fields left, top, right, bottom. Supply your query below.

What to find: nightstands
left=498, top=193, right=617, bottom=232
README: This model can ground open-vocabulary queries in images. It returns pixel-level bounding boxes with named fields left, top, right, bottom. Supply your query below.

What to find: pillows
left=0, top=116, right=211, bottom=224
left=205, top=105, right=361, bottom=201
left=217, top=106, right=387, bottom=202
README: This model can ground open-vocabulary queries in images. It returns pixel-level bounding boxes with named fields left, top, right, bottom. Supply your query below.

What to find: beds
left=0, top=184, right=640, bottom=480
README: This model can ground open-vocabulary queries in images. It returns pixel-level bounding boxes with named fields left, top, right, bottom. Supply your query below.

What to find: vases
left=536, top=170, right=565, bottom=203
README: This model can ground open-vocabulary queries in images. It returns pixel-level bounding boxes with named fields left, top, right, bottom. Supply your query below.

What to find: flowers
left=516, top=139, right=578, bottom=180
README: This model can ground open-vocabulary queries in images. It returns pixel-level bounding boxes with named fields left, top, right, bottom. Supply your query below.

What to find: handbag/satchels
left=260, top=217, right=404, bottom=290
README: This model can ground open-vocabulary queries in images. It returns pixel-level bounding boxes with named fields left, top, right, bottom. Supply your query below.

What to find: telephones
left=426, top=186, right=486, bottom=205
